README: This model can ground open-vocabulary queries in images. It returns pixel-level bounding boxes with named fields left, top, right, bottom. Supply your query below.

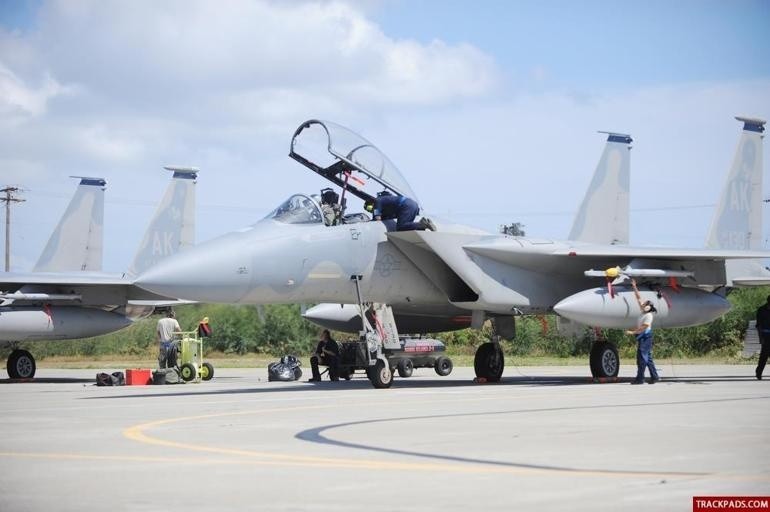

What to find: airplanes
left=0, top=115, right=770, bottom=388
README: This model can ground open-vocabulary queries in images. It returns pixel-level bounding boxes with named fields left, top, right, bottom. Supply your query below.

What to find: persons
left=308, top=330, right=341, bottom=382
left=755, top=295, right=770, bottom=379
left=155, top=309, right=184, bottom=369
left=625, top=279, right=662, bottom=385
left=364, top=195, right=440, bottom=232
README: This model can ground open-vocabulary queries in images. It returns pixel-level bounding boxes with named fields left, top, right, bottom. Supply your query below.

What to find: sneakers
left=631, top=379, right=644, bottom=384
left=756, top=367, right=762, bottom=380
left=331, top=378, right=339, bottom=381
left=309, top=379, right=321, bottom=382
left=648, top=377, right=661, bottom=384
left=420, top=217, right=437, bottom=231
